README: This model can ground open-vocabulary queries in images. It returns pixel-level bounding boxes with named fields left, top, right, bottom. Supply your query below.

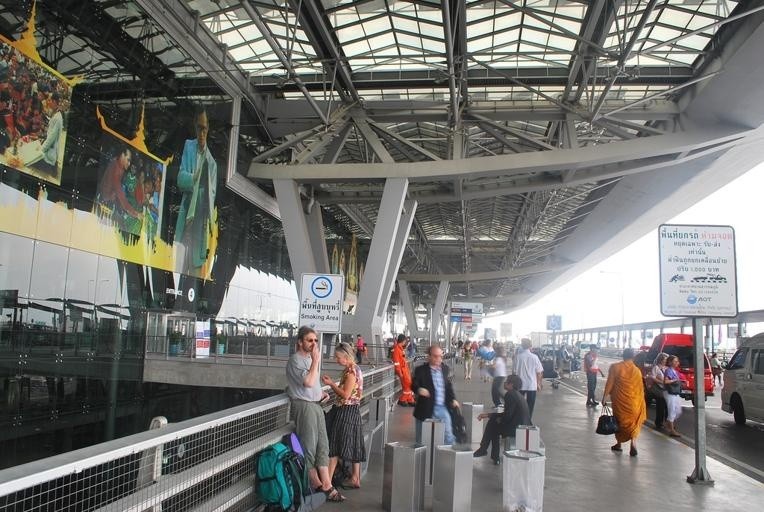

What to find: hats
left=590, top=345, right=599, bottom=349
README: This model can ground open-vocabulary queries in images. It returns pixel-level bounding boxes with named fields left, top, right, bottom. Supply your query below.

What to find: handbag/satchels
left=650, top=382, right=681, bottom=399
left=595, top=406, right=619, bottom=434
left=452, top=408, right=466, bottom=439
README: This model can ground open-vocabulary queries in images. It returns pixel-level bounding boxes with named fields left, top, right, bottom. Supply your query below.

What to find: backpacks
left=253, top=441, right=311, bottom=512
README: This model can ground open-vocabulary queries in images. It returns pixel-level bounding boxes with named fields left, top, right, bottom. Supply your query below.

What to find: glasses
left=302, top=337, right=319, bottom=344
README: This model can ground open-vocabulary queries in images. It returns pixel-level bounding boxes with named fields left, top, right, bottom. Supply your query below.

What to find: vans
left=720, top=333, right=764, bottom=429
left=578, top=341, right=595, bottom=359
left=645, top=333, right=715, bottom=405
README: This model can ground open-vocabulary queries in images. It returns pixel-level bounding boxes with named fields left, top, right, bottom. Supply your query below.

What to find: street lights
left=86, top=279, right=94, bottom=318
left=97, top=280, right=109, bottom=301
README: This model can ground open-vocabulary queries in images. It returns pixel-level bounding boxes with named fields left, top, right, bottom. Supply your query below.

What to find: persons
left=711, top=353, right=722, bottom=386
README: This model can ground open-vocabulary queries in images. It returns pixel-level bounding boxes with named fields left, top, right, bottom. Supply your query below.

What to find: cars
left=541, top=346, right=559, bottom=368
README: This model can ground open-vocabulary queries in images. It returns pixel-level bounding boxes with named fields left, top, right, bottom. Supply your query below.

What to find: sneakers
left=473, top=446, right=487, bottom=457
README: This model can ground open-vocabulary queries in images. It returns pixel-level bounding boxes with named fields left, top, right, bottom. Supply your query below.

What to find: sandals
left=314, top=475, right=361, bottom=502
